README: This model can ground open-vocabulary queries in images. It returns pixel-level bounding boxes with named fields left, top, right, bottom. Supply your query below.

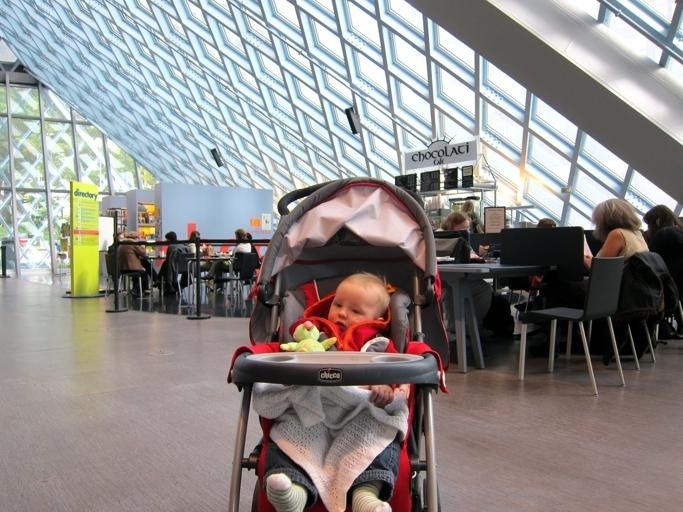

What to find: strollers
left=225, top=176, right=452, bottom=511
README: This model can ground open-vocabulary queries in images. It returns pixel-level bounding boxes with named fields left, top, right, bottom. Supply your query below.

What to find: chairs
left=106, top=247, right=251, bottom=318
left=518, top=256, right=627, bottom=395
left=566, top=251, right=662, bottom=371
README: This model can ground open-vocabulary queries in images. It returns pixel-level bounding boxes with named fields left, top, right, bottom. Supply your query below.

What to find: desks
left=437, top=263, right=557, bottom=374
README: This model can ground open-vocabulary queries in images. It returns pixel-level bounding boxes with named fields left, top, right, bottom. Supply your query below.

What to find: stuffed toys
left=280, top=319, right=337, bottom=352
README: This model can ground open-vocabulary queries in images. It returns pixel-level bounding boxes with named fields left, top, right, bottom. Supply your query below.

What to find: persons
left=643, top=205, right=682, bottom=300
left=462, top=201, right=485, bottom=234
left=582, top=200, right=649, bottom=365
left=435, top=211, right=473, bottom=235
left=181, top=230, right=261, bottom=291
left=151, top=231, right=188, bottom=295
left=256, top=272, right=405, bottom=512
left=536, top=218, right=558, bottom=228
left=106, top=231, right=160, bottom=293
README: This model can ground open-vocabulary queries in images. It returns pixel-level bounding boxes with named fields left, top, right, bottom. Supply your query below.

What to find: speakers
left=345, top=108, right=361, bottom=134
left=211, top=149, right=223, bottom=167
